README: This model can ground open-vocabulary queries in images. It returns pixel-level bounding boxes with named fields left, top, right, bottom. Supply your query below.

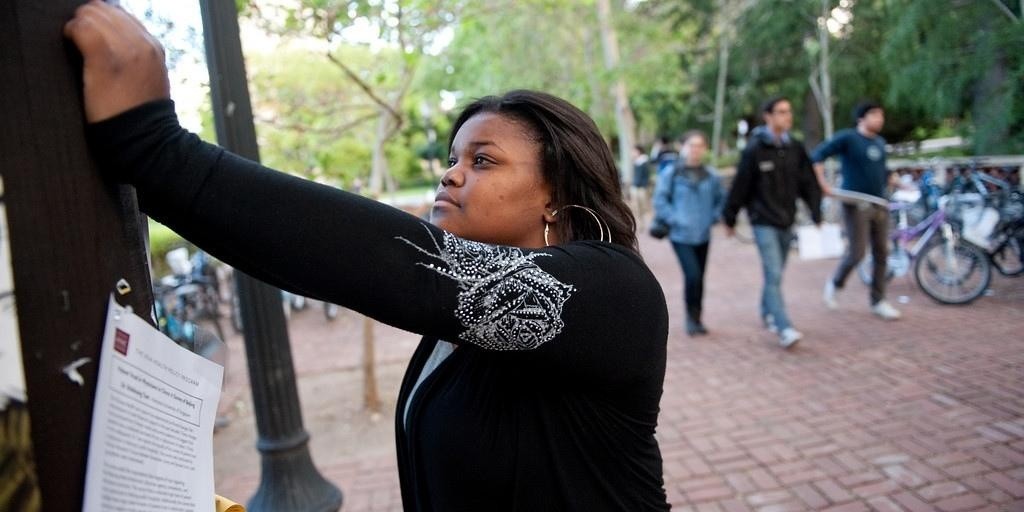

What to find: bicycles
left=852, top=158, right=1023, bottom=307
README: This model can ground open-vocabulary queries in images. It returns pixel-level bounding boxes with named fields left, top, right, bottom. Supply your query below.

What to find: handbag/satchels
left=650, top=219, right=669, bottom=239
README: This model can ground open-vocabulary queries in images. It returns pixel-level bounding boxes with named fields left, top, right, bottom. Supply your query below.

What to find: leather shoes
left=688, top=315, right=705, bottom=335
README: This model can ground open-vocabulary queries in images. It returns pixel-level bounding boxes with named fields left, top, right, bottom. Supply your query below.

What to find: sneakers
left=869, top=298, right=901, bottom=320
left=824, top=278, right=840, bottom=309
left=762, top=310, right=804, bottom=348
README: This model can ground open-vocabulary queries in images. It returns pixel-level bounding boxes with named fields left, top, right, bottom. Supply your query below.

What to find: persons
left=63, top=1, right=670, bottom=512
left=807, top=103, right=908, bottom=320
left=652, top=130, right=727, bottom=336
left=630, top=145, right=654, bottom=228
left=720, top=100, right=824, bottom=349
